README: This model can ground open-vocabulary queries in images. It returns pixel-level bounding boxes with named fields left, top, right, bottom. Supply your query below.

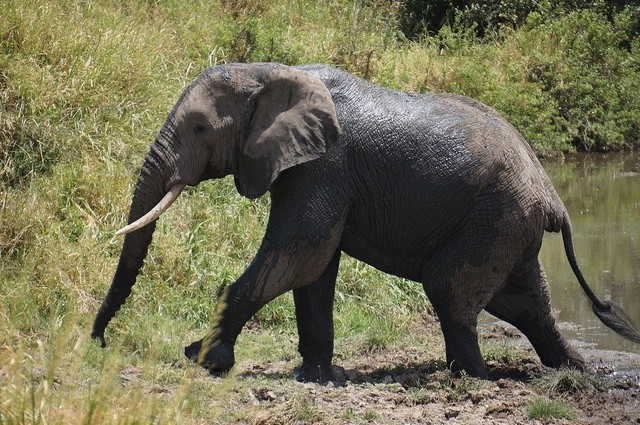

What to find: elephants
left=91, top=62, right=640, bottom=381
